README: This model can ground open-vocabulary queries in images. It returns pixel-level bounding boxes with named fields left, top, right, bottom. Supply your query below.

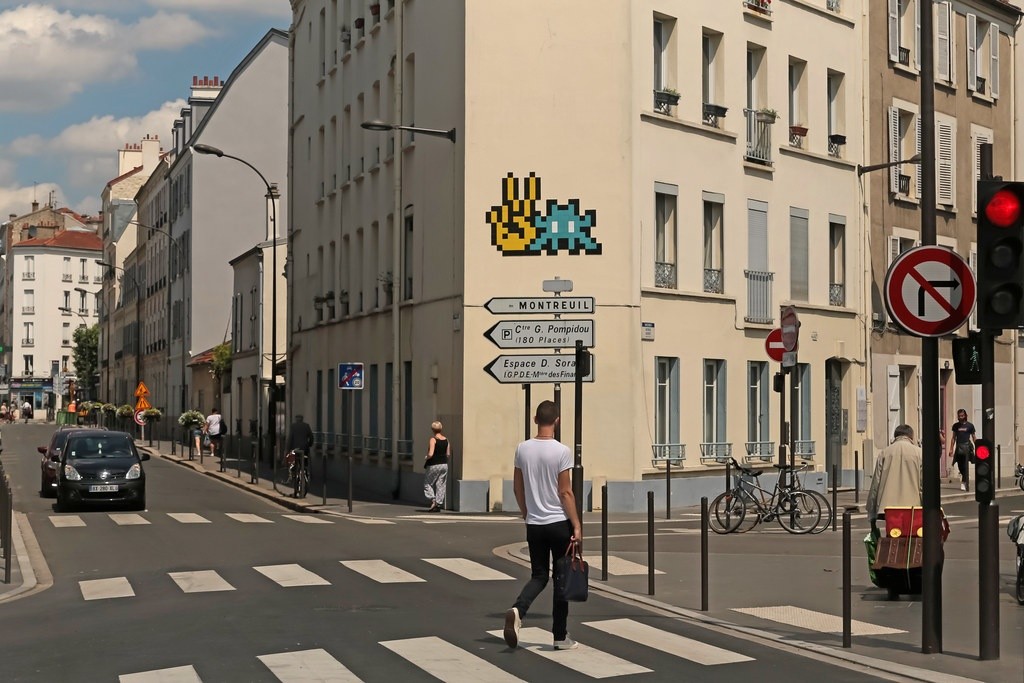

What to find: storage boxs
left=884, top=505, right=951, bottom=542
left=871, top=536, right=923, bottom=588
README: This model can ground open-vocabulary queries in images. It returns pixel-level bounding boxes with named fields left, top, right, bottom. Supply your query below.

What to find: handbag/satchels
left=864, top=522, right=888, bottom=588
left=561, top=541, right=589, bottom=602
left=204, top=437, right=211, bottom=447
left=218, top=418, right=228, bottom=435
left=285, top=451, right=296, bottom=470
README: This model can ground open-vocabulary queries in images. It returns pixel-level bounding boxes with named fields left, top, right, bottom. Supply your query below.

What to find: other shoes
left=196, top=452, right=201, bottom=456
left=210, top=453, right=214, bottom=457
left=428, top=501, right=441, bottom=512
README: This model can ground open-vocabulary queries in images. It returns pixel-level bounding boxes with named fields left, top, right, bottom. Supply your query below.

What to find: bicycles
left=715, top=460, right=833, bottom=534
left=273, top=449, right=310, bottom=498
left=706, top=457, right=822, bottom=535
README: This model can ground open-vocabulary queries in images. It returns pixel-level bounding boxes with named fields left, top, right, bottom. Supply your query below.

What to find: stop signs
left=765, top=330, right=799, bottom=363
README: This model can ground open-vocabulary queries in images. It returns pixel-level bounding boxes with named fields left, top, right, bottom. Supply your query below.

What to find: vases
left=704, top=103, right=728, bottom=117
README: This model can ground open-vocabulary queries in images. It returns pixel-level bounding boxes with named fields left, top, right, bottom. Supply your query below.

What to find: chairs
left=75, top=439, right=94, bottom=456
left=106, top=437, right=124, bottom=451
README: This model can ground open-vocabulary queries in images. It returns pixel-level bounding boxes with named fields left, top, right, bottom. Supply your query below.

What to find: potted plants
left=829, top=133, right=847, bottom=146
left=325, top=290, right=335, bottom=308
left=789, top=122, right=809, bottom=136
left=655, top=87, right=682, bottom=106
left=79, top=400, right=92, bottom=412
left=90, top=402, right=103, bottom=413
left=313, top=295, right=325, bottom=310
left=757, top=107, right=782, bottom=124
left=140, top=407, right=163, bottom=423
left=339, top=289, right=349, bottom=304
left=177, top=408, right=207, bottom=430
left=376, top=270, right=394, bottom=292
left=100, top=402, right=117, bottom=417
left=115, top=404, right=135, bottom=424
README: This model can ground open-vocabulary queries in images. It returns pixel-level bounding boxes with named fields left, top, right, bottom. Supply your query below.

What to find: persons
left=0, top=399, right=32, bottom=424
left=285, top=415, right=314, bottom=486
left=866, top=409, right=976, bottom=598
left=504, top=402, right=583, bottom=650
left=194, top=407, right=223, bottom=456
left=423, top=421, right=451, bottom=512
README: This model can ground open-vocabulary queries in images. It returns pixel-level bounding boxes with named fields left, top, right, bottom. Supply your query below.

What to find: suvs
left=36, top=426, right=111, bottom=498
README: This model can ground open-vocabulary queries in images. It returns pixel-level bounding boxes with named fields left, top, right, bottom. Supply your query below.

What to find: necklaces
left=537, top=434, right=553, bottom=438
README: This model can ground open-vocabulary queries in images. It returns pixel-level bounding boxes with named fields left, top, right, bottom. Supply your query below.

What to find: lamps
left=857, top=152, right=922, bottom=177
left=361, top=118, right=458, bottom=144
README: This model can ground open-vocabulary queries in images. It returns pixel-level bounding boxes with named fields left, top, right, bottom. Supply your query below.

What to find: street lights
left=130, top=218, right=187, bottom=444
left=95, top=259, right=140, bottom=433
left=57, top=306, right=89, bottom=402
left=75, top=286, right=110, bottom=405
left=192, top=142, right=279, bottom=470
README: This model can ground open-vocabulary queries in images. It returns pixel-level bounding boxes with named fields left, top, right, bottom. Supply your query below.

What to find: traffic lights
left=972, top=438, right=996, bottom=504
left=952, top=336, right=983, bottom=386
left=974, top=178, right=1023, bottom=329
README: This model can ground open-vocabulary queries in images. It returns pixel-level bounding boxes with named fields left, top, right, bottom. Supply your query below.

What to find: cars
left=51, top=429, right=150, bottom=512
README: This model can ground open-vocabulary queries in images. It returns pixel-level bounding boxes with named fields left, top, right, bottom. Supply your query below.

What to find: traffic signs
left=482, top=319, right=595, bottom=349
left=485, top=295, right=596, bottom=314
left=482, top=352, right=596, bottom=384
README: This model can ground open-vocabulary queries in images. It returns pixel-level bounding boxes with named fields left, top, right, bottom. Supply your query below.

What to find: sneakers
left=504, top=607, right=522, bottom=648
left=553, top=637, right=578, bottom=649
